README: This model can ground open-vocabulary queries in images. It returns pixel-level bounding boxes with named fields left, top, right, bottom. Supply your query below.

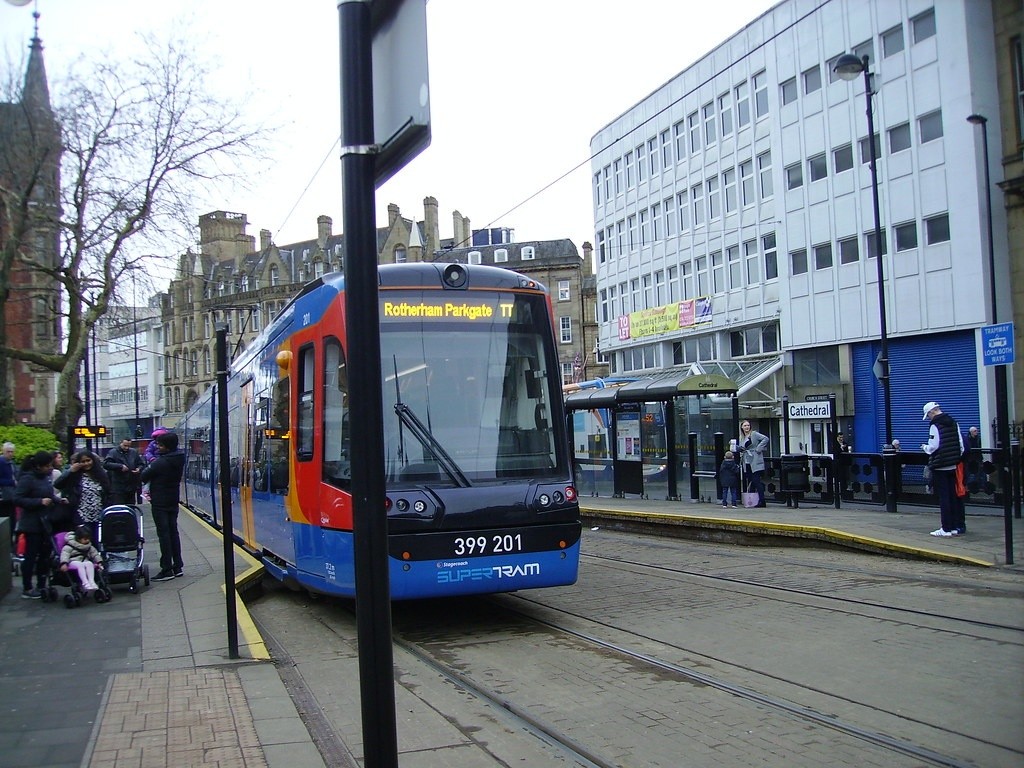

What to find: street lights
left=833, top=54, right=898, bottom=513
left=969, top=114, right=1006, bottom=498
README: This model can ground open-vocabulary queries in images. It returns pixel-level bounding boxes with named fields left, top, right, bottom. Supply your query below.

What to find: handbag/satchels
left=741, top=482, right=759, bottom=507
left=954, top=461, right=965, bottom=498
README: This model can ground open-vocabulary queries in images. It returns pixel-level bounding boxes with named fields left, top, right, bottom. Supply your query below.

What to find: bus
left=170, top=261, right=582, bottom=595
left=561, top=376, right=667, bottom=482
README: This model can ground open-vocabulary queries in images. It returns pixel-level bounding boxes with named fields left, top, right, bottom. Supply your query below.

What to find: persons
left=230, top=457, right=289, bottom=490
left=0, top=428, right=186, bottom=599
left=719, top=451, right=740, bottom=508
left=739, top=420, right=769, bottom=508
left=836, top=432, right=849, bottom=451
left=964, top=427, right=984, bottom=462
left=920, top=402, right=966, bottom=536
left=892, top=439, right=901, bottom=451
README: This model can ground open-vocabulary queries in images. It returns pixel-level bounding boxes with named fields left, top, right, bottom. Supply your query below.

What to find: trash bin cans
left=780, top=453, right=810, bottom=494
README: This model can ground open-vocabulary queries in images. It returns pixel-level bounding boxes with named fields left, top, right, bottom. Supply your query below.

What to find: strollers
left=40, top=506, right=112, bottom=607
left=97, top=505, right=150, bottom=594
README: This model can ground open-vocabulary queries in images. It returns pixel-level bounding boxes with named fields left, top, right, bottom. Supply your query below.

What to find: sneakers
left=930, top=528, right=952, bottom=537
left=21, top=588, right=42, bottom=600
left=150, top=570, right=175, bottom=581
left=35, top=586, right=45, bottom=593
left=951, top=529, right=958, bottom=535
left=171, top=568, right=183, bottom=577
left=140, top=490, right=151, bottom=500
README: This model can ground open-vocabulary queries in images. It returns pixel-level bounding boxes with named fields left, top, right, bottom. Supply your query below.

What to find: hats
left=923, top=402, right=939, bottom=420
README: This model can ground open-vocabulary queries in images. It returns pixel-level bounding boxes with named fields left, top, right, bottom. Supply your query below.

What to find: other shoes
left=82, top=581, right=93, bottom=590
left=732, top=505, right=737, bottom=509
left=754, top=501, right=766, bottom=507
left=90, top=582, right=99, bottom=590
left=722, top=505, right=728, bottom=509
